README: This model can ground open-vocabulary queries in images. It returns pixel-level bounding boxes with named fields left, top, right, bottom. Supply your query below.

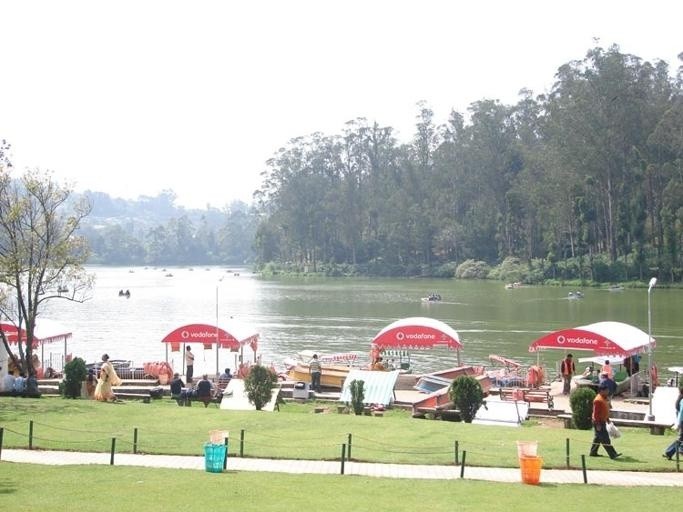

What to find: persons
left=560, top=353, right=575, bottom=397
left=193, top=373, right=213, bottom=401
left=85, top=369, right=97, bottom=399
left=216, top=368, right=233, bottom=401
left=308, top=354, right=322, bottom=394
left=627, top=357, right=639, bottom=378
left=184, top=345, right=195, bottom=384
left=98, top=354, right=117, bottom=403
left=579, top=366, right=594, bottom=380
left=169, top=372, right=185, bottom=400
left=599, top=360, right=614, bottom=380
left=378, top=357, right=384, bottom=368
left=662, top=398, right=683, bottom=460
left=590, top=368, right=600, bottom=384
left=373, top=357, right=380, bottom=370
left=675, top=385, right=683, bottom=416
left=595, top=372, right=617, bottom=399
left=7, top=353, right=63, bottom=396
left=589, top=383, right=622, bottom=459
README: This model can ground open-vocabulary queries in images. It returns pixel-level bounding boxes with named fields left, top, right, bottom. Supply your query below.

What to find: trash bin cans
left=208, top=429, right=229, bottom=444
left=517, top=440, right=537, bottom=457
left=204, top=442, right=227, bottom=473
left=520, top=455, right=543, bottom=484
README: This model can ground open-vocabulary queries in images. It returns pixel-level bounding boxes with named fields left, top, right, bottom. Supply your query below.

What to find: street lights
left=647, top=276, right=657, bottom=421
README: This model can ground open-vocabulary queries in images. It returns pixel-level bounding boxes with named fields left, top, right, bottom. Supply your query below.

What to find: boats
left=284, top=315, right=492, bottom=398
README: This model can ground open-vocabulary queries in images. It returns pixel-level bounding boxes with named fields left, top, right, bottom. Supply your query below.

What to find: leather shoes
left=590, top=453, right=623, bottom=458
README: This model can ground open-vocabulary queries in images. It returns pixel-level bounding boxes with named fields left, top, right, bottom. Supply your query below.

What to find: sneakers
left=663, top=454, right=671, bottom=460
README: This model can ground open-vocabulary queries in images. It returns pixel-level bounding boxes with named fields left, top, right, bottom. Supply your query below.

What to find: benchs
left=172, top=395, right=221, bottom=408
left=499, top=387, right=555, bottom=408
left=557, top=413, right=674, bottom=435
left=0, top=390, right=42, bottom=398
left=417, top=407, right=461, bottom=420
left=115, top=393, right=151, bottom=403
